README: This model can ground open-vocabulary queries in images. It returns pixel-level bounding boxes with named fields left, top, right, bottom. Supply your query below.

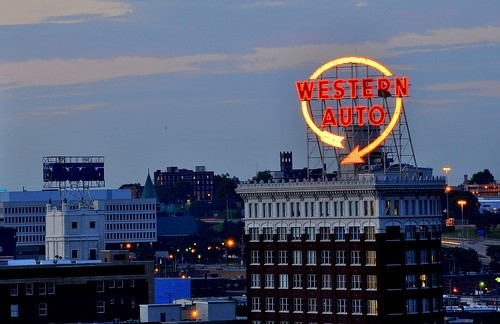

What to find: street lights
left=480, top=281, right=484, bottom=305
left=442, top=167, right=451, bottom=186
left=458, top=200, right=467, bottom=249
left=445, top=187, right=451, bottom=219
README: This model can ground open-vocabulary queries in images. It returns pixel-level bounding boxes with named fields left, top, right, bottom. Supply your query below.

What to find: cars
left=442, top=293, right=500, bottom=313
